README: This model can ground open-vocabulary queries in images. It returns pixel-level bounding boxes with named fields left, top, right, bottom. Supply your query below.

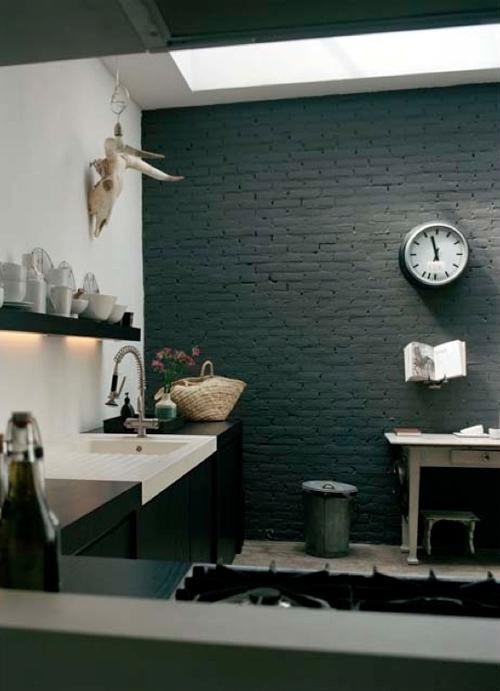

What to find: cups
left=0, top=262, right=72, bottom=319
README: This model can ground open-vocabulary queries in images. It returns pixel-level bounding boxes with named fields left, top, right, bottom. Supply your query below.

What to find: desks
left=384, top=426, right=500, bottom=567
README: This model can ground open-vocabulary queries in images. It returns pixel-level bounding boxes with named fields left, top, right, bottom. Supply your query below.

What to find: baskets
left=153, top=360, right=247, bottom=422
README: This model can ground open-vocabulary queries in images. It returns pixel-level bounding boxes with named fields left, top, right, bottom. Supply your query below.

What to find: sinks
left=68, top=439, right=187, bottom=456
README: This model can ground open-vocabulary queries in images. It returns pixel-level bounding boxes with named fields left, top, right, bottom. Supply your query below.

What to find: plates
left=30, top=247, right=100, bottom=293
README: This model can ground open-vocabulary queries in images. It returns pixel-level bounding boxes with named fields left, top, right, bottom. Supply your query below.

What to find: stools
left=423, top=508, right=480, bottom=558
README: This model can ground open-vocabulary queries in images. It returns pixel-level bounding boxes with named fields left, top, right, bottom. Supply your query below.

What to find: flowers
left=150, top=343, right=203, bottom=390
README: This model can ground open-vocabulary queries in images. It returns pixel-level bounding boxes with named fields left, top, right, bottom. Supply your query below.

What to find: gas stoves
left=172, top=561, right=500, bottom=620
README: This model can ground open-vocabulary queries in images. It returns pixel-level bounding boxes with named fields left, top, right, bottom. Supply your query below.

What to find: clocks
left=399, top=220, right=471, bottom=292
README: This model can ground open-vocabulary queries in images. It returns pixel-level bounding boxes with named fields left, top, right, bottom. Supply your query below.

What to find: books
left=403, top=338, right=469, bottom=385
left=394, top=427, right=422, bottom=436
left=453, top=432, right=489, bottom=438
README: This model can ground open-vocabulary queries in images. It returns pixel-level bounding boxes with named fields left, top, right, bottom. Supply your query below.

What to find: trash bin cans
left=301, top=480, right=359, bottom=558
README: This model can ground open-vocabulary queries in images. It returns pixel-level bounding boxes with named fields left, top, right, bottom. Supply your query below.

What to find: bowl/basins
left=72, top=293, right=129, bottom=325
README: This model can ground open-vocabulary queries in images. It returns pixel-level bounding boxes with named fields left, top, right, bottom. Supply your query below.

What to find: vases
left=153, top=392, right=180, bottom=422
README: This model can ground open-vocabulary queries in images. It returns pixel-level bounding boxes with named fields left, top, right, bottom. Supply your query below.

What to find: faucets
left=105, top=345, right=146, bottom=437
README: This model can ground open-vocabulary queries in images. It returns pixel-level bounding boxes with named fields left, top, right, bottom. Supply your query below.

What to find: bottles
left=1, top=412, right=60, bottom=593
